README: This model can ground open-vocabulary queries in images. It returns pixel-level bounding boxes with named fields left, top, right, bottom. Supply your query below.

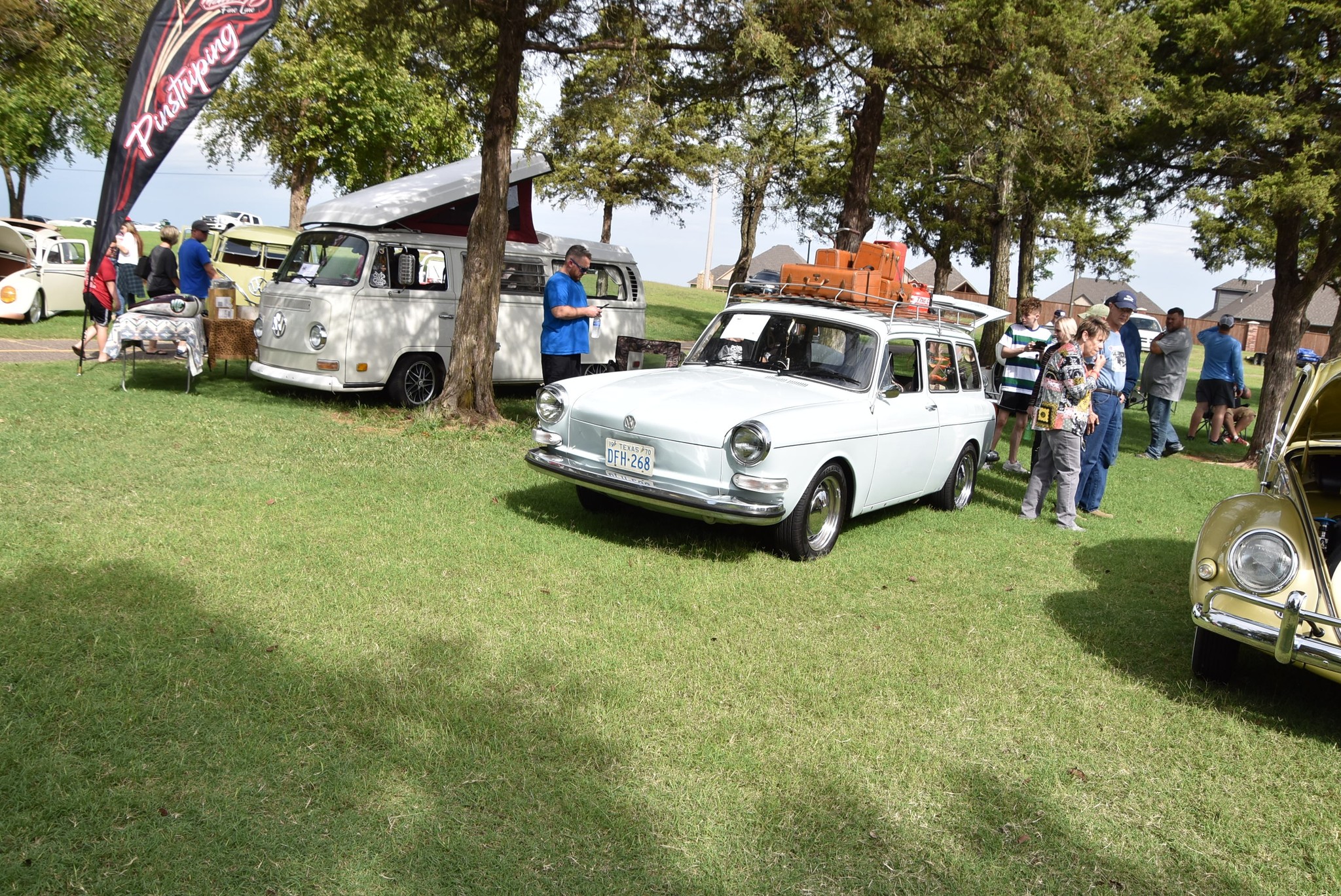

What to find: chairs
left=763, top=340, right=813, bottom=378
left=607, top=336, right=681, bottom=372
left=839, top=347, right=905, bottom=391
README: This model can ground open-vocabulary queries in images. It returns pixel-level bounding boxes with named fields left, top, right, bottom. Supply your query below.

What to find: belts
left=1093, top=387, right=1123, bottom=397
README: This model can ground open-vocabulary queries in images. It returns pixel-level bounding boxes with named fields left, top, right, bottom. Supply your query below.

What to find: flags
left=86, top=0, right=281, bottom=276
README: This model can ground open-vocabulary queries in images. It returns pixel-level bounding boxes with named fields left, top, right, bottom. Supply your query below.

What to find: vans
left=249, top=149, right=647, bottom=409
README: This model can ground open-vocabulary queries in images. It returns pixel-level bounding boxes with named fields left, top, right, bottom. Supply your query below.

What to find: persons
left=1018, top=314, right=1111, bottom=530
left=1074, top=290, right=1133, bottom=521
left=1076, top=303, right=1110, bottom=321
left=1184, top=314, right=1244, bottom=445
left=146, top=224, right=181, bottom=355
left=174, top=219, right=220, bottom=359
left=704, top=312, right=767, bottom=371
left=1044, top=310, right=1066, bottom=326
left=1222, top=382, right=1255, bottom=447
left=115, top=216, right=146, bottom=317
left=72, top=236, right=121, bottom=362
left=1136, top=307, right=1192, bottom=461
left=540, top=245, right=602, bottom=385
left=926, top=341, right=980, bottom=391
left=981, top=298, right=1053, bottom=474
left=1026, top=315, right=1078, bottom=477
left=1105, top=296, right=1141, bottom=411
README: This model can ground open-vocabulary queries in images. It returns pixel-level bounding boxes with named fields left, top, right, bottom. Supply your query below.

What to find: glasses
left=109, top=246, right=116, bottom=251
left=570, top=258, right=589, bottom=273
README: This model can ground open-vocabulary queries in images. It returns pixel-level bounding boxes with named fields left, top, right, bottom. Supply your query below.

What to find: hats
left=1054, top=310, right=1066, bottom=317
left=1077, top=304, right=1110, bottom=320
left=1219, top=314, right=1234, bottom=329
left=1111, top=290, right=1136, bottom=311
left=192, top=220, right=209, bottom=231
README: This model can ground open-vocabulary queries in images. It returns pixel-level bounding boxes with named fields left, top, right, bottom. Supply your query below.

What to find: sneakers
left=982, top=462, right=994, bottom=471
left=1208, top=439, right=1223, bottom=446
left=1183, top=436, right=1195, bottom=441
left=1233, top=437, right=1249, bottom=447
left=1052, top=506, right=1114, bottom=532
left=1223, top=434, right=1234, bottom=445
left=1002, top=460, right=1031, bottom=475
left=1135, top=448, right=1183, bottom=460
left=173, top=348, right=188, bottom=359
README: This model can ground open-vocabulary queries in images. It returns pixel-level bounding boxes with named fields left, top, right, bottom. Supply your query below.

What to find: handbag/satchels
left=133, top=255, right=152, bottom=280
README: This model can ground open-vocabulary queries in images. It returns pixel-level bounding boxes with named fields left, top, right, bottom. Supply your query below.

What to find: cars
left=1187, top=352, right=1341, bottom=694
left=0, top=210, right=275, bottom=324
left=1129, top=312, right=1169, bottom=352
left=1244, top=348, right=1323, bottom=369
left=175, top=225, right=322, bottom=311
left=741, top=269, right=781, bottom=296
left=525, top=281, right=1012, bottom=562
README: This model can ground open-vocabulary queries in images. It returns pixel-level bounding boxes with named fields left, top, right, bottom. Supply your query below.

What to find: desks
left=202, top=317, right=257, bottom=381
left=102, top=312, right=206, bottom=394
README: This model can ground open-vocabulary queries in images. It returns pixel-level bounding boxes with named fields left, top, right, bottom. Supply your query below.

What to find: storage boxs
left=208, top=288, right=237, bottom=320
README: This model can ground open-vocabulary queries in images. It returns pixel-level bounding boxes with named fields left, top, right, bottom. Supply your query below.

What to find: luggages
left=779, top=240, right=912, bottom=308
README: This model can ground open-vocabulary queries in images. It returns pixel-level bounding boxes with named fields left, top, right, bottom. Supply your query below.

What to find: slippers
left=72, top=344, right=169, bottom=363
